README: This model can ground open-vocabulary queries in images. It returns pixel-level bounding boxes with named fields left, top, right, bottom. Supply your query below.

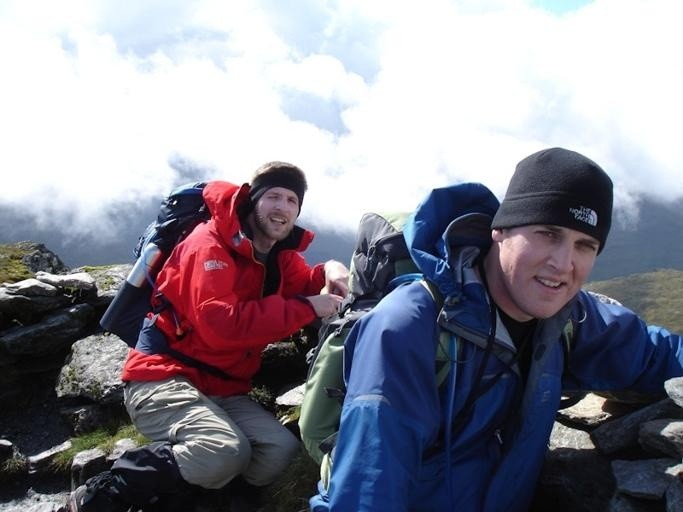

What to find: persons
left=309, top=147, right=683, bottom=512
left=67, top=161, right=351, bottom=512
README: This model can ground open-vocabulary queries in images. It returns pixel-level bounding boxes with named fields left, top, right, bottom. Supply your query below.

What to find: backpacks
left=99, top=182, right=237, bottom=350
left=299, top=211, right=579, bottom=469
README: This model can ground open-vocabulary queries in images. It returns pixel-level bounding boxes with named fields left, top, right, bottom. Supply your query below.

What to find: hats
left=248, top=161, right=307, bottom=217
left=490, top=147, right=613, bottom=256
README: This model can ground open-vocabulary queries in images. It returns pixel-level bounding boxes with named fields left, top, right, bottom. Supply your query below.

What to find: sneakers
left=70, top=483, right=87, bottom=512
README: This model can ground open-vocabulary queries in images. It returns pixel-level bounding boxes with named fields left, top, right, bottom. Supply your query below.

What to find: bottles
left=100, top=241, right=163, bottom=333
